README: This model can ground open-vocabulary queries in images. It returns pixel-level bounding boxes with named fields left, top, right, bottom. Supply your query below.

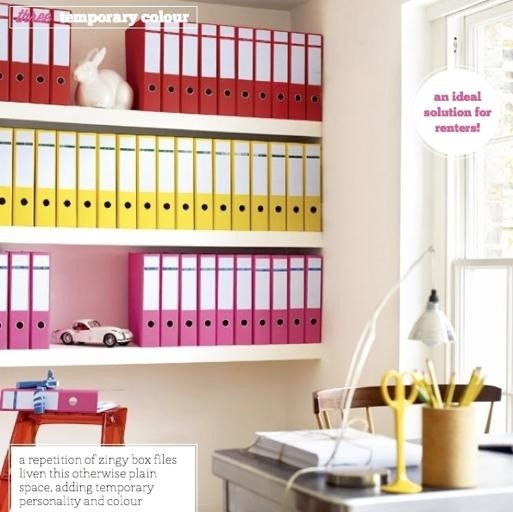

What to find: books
left=247, top=427, right=423, bottom=469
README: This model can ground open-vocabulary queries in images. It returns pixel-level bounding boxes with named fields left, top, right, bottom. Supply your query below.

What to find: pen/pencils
left=413, top=357, right=487, bottom=409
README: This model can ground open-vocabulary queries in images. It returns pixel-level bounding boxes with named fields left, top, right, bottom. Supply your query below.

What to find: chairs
left=312, top=384, right=502, bottom=434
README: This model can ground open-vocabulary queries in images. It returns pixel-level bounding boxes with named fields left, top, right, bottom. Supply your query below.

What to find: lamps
left=340, top=246, right=458, bottom=430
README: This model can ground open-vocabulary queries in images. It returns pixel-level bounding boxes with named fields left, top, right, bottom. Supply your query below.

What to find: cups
left=422, top=404, right=475, bottom=489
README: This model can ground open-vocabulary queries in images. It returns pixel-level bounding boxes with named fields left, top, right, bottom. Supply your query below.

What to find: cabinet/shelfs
left=212, top=434, right=513, bottom=512
left=0, top=100, right=324, bottom=368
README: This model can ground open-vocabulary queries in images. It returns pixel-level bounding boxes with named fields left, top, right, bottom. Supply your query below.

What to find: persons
left=53, top=318, right=135, bottom=346
